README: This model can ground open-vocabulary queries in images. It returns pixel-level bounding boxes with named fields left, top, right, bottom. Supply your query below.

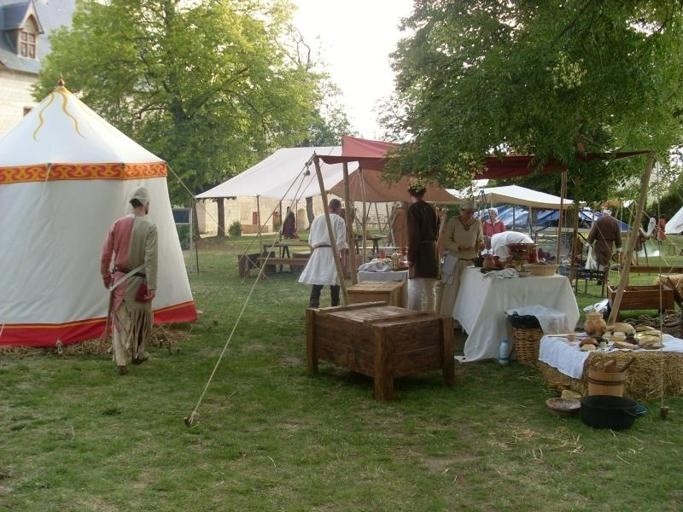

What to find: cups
left=660, top=406, right=667, bottom=418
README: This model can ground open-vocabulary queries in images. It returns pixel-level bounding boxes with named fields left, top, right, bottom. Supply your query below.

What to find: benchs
left=256, top=255, right=310, bottom=277
left=238, top=250, right=274, bottom=278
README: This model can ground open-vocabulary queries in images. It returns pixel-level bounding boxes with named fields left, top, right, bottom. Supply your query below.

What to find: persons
left=482, top=207, right=505, bottom=249
left=98, top=187, right=158, bottom=375
left=586, top=211, right=622, bottom=286
left=281, top=206, right=300, bottom=239
left=406, top=184, right=440, bottom=312
left=656, top=214, right=667, bottom=247
left=439, top=200, right=484, bottom=333
left=298, top=199, right=345, bottom=308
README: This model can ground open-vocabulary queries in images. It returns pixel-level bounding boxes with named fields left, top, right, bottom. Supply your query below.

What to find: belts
left=114, top=267, right=146, bottom=278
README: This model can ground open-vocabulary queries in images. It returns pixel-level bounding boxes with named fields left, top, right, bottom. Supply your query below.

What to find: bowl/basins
left=582, top=394, right=636, bottom=428
left=470, top=258, right=483, bottom=266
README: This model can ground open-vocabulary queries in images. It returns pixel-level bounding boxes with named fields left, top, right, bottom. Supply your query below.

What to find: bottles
left=390, top=249, right=408, bottom=271
left=482, top=256, right=494, bottom=268
left=583, top=313, right=604, bottom=337
left=499, top=338, right=509, bottom=365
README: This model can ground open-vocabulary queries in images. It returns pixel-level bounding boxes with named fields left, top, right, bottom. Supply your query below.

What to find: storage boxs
left=606, top=285, right=673, bottom=311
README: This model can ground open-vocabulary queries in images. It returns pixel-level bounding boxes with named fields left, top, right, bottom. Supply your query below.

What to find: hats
left=460, top=199, right=475, bottom=210
left=488, top=207, right=498, bottom=216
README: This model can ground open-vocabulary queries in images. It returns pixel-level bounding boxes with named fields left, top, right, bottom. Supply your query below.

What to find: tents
left=663, top=203, right=683, bottom=234
left=472, top=204, right=632, bottom=234
left=0, top=71, right=197, bottom=353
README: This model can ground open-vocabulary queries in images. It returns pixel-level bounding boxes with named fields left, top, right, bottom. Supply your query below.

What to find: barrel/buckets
left=584, top=349, right=627, bottom=399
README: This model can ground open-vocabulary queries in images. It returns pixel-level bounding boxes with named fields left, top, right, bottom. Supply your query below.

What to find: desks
left=355, top=257, right=408, bottom=307
left=260, top=239, right=308, bottom=273
left=452, top=264, right=582, bottom=363
left=303, top=299, right=454, bottom=401
left=347, top=279, right=404, bottom=307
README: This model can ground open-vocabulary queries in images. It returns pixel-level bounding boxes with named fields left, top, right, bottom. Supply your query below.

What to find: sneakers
left=118, top=351, right=149, bottom=376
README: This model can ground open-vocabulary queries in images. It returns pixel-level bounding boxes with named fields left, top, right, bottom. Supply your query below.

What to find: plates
left=546, top=397, right=578, bottom=411
left=637, top=405, right=647, bottom=415
